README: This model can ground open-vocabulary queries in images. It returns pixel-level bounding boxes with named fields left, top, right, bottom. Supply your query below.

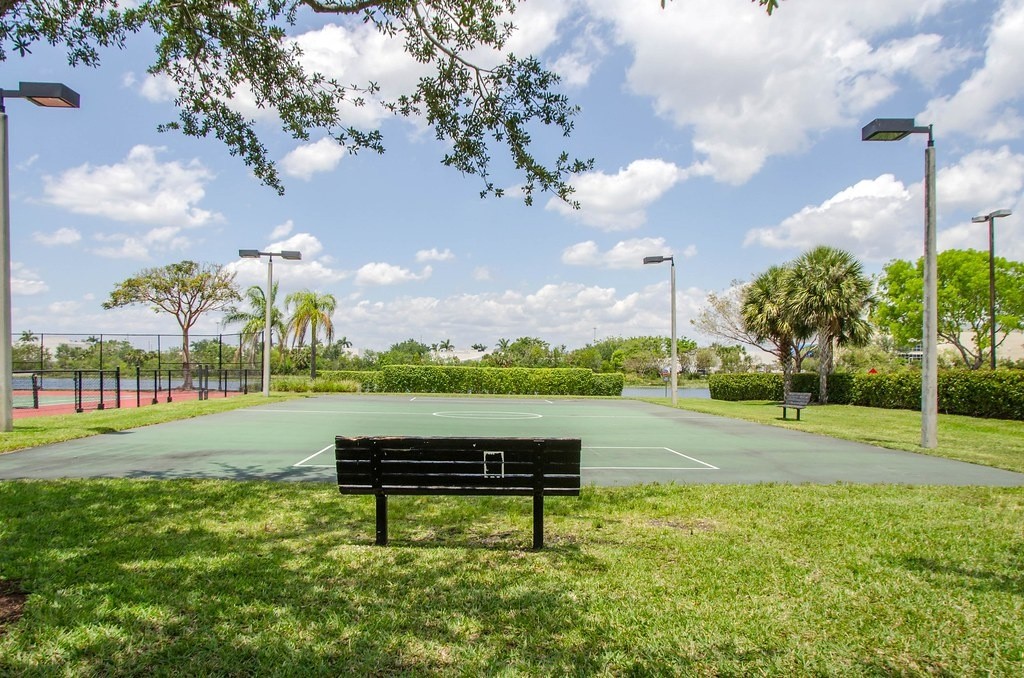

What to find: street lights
left=862, top=119, right=937, bottom=447
left=239, top=249, right=302, bottom=397
left=641, top=254, right=677, bottom=407
left=970, top=211, right=1008, bottom=373
left=0, top=81, right=81, bottom=423
left=593, top=327, right=596, bottom=347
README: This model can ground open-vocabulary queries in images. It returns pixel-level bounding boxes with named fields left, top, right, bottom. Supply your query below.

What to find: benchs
left=335, top=434, right=582, bottom=547
left=777, top=393, right=811, bottom=420
